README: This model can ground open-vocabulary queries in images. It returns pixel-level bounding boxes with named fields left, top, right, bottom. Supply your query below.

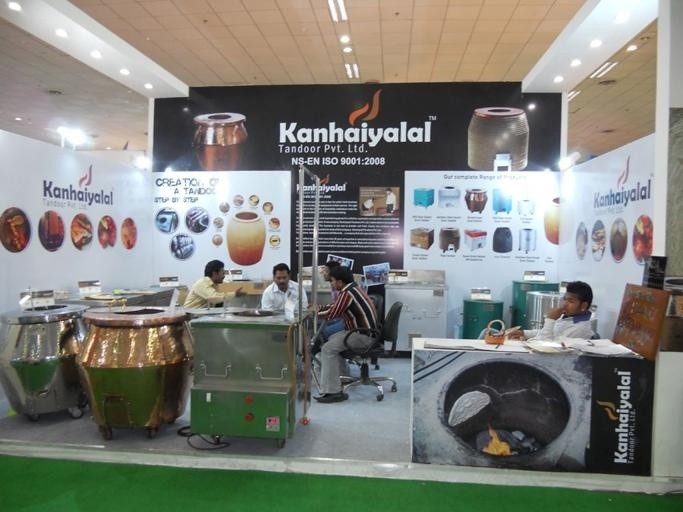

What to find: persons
left=181, top=260, right=247, bottom=308
left=384, top=188, right=396, bottom=214
left=361, top=198, right=374, bottom=211
left=506, top=280, right=594, bottom=342
left=260, top=263, right=309, bottom=311
left=312, top=265, right=377, bottom=403
left=304, top=261, right=347, bottom=359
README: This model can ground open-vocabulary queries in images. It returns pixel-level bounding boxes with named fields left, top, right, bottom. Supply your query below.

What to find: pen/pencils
left=561, top=342, right=565, bottom=347
left=495, top=343, right=501, bottom=349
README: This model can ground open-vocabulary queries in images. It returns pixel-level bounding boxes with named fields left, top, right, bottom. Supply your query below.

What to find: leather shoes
left=318, top=393, right=348, bottom=403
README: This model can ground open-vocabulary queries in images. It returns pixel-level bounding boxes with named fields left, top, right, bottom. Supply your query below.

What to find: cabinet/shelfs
left=382, top=290, right=448, bottom=353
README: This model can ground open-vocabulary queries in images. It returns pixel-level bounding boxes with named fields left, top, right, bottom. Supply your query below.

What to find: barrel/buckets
left=523, top=292, right=566, bottom=330
left=463, top=300, right=504, bottom=339
left=512, top=280, right=559, bottom=330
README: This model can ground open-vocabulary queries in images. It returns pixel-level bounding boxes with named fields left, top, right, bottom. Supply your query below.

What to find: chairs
left=336, top=294, right=403, bottom=401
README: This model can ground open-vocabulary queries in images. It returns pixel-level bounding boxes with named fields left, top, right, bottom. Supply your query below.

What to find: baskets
left=485, top=320, right=505, bottom=344
